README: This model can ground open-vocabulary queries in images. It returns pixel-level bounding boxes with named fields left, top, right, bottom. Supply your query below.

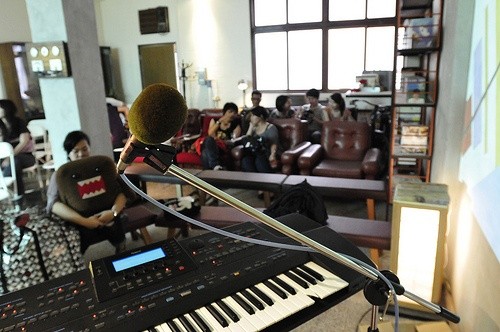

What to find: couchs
left=232, top=117, right=308, bottom=175
left=175, top=114, right=245, bottom=170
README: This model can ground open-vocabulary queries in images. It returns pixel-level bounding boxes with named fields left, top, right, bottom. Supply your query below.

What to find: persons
left=242, top=90, right=270, bottom=134
left=400, top=67, right=426, bottom=91
left=203, top=102, right=241, bottom=170
left=408, top=88, right=424, bottom=104
left=107, top=103, right=129, bottom=163
left=47, top=131, right=131, bottom=255
left=323, top=92, right=355, bottom=121
left=0, top=99, right=36, bottom=209
left=242, top=107, right=279, bottom=174
left=296, top=89, right=323, bottom=144
left=271, top=95, right=296, bottom=119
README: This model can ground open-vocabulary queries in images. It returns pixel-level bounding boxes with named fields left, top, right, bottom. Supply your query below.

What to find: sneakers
left=214, top=165, right=224, bottom=170
left=112, top=237, right=129, bottom=255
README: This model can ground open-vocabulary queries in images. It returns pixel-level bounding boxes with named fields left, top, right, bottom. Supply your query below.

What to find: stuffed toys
left=56, top=155, right=119, bottom=219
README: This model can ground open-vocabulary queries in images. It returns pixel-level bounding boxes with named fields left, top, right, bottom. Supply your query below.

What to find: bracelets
left=215, top=121, right=221, bottom=124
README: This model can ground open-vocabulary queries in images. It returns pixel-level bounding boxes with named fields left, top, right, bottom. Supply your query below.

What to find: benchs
left=283, top=175, right=386, bottom=220
left=125, top=163, right=203, bottom=198
left=156, top=205, right=266, bottom=239
left=325, top=214, right=391, bottom=267
left=196, top=169, right=287, bottom=207
left=122, top=205, right=163, bottom=245
left=298, top=109, right=381, bottom=180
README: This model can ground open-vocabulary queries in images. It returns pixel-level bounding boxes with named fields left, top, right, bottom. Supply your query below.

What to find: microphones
left=116, top=83, right=188, bottom=177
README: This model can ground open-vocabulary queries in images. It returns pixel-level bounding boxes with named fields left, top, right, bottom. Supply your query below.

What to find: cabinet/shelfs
left=388, top=0, right=444, bottom=203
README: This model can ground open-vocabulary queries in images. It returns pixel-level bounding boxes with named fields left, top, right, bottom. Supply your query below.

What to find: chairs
left=0, top=119, right=57, bottom=214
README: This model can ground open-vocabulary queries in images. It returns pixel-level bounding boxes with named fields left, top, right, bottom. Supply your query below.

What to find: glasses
left=251, top=98, right=260, bottom=100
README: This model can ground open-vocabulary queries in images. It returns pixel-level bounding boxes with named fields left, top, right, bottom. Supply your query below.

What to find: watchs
left=111, top=209, right=117, bottom=217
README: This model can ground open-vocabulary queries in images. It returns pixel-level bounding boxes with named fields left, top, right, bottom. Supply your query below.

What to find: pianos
left=0, top=212, right=380, bottom=332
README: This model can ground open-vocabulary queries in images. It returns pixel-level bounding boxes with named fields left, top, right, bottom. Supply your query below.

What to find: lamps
left=237, top=79, right=248, bottom=107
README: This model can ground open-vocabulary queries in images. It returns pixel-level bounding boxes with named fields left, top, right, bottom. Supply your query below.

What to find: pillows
left=57, top=155, right=121, bottom=215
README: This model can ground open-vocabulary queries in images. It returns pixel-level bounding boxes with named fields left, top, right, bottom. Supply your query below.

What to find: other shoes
left=270, top=194, right=275, bottom=201
left=257, top=192, right=264, bottom=199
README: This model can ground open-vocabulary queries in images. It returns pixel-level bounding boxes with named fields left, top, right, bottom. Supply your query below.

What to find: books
left=394, top=124, right=429, bottom=156
left=398, top=156, right=417, bottom=165
